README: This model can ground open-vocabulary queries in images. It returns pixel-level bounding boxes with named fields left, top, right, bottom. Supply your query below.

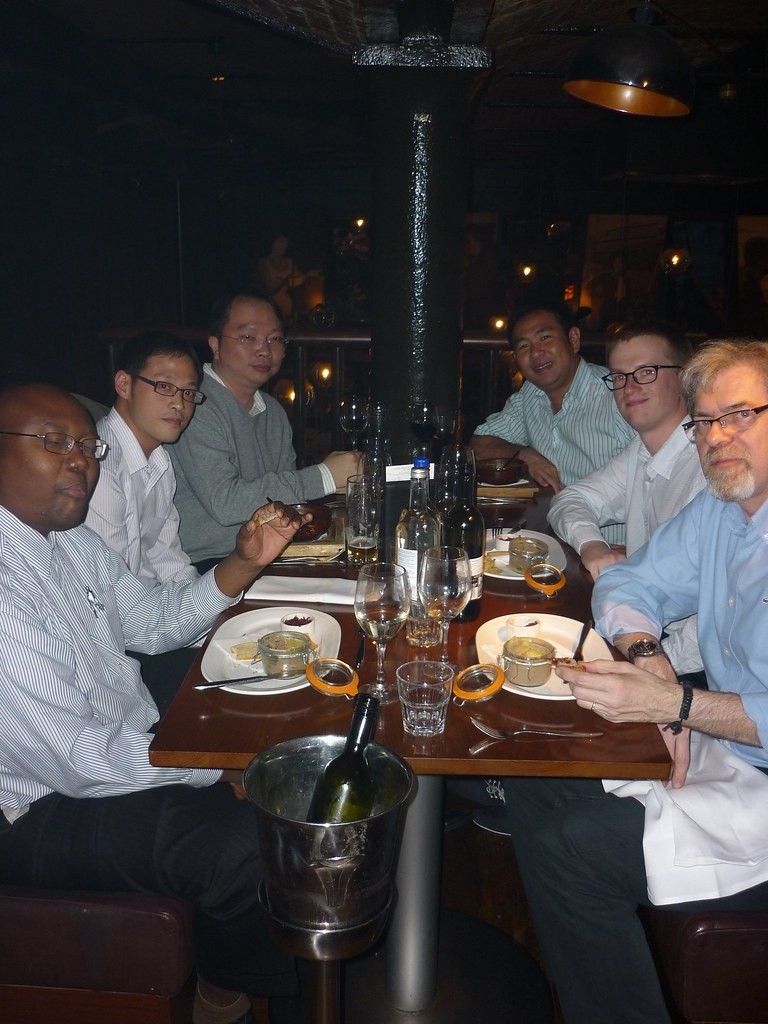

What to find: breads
left=231, top=643, right=262, bottom=660
left=253, top=512, right=277, bottom=527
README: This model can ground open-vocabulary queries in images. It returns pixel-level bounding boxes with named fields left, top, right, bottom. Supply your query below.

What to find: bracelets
left=662, top=681, right=693, bottom=735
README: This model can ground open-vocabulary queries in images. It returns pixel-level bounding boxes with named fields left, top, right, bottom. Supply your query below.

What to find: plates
left=481, top=528, right=567, bottom=580
left=200, top=604, right=342, bottom=695
left=474, top=610, right=615, bottom=702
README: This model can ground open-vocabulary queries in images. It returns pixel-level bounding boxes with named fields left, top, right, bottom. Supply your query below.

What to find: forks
left=492, top=515, right=506, bottom=539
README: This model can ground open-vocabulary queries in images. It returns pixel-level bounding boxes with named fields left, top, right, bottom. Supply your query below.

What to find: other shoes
left=470, top=806, right=512, bottom=836
left=454, top=774, right=505, bottom=807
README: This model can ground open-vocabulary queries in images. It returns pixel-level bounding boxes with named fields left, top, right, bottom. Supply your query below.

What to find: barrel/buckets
left=239, top=732, right=414, bottom=932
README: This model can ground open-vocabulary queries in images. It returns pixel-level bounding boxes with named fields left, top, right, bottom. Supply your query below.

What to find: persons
left=739, top=236, right=768, bottom=338
left=0, top=382, right=313, bottom=1024
left=95, top=329, right=207, bottom=647
left=472, top=319, right=710, bottom=836
left=469, top=304, right=638, bottom=544
left=163, top=280, right=364, bottom=575
left=504, top=336, right=768, bottom=1024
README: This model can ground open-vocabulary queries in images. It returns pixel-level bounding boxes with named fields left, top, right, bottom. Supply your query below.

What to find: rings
left=591, top=702, right=595, bottom=711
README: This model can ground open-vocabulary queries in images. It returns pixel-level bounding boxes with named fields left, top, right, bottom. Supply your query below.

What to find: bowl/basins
left=508, top=536, right=549, bottom=572
left=499, top=635, right=555, bottom=686
left=286, top=501, right=334, bottom=542
left=476, top=456, right=525, bottom=484
left=257, top=632, right=315, bottom=679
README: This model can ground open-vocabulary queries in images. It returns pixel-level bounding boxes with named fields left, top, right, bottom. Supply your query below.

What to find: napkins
left=245, top=574, right=384, bottom=605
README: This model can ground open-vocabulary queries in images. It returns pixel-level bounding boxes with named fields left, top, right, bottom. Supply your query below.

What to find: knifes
left=469, top=717, right=606, bottom=741
left=506, top=518, right=527, bottom=536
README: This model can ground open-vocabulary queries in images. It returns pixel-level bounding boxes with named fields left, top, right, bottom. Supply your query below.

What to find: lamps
left=561, top=5, right=696, bottom=116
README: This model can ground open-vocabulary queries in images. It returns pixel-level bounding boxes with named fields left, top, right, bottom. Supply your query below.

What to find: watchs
left=627, top=638, right=665, bottom=665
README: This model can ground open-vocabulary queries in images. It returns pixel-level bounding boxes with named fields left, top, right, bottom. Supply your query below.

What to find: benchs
left=0, top=875, right=201, bottom=1024
left=635, top=904, right=768, bottom=1024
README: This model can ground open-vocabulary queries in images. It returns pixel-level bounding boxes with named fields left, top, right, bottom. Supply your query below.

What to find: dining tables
left=148, top=449, right=673, bottom=1024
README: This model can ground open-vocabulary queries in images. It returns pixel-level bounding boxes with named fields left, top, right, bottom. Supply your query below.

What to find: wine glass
left=339, top=396, right=474, bottom=511
left=354, top=566, right=403, bottom=707
left=416, top=545, right=463, bottom=680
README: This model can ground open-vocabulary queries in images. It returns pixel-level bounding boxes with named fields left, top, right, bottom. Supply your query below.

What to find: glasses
left=0, top=431, right=110, bottom=459
left=682, top=405, right=768, bottom=442
left=127, top=371, right=206, bottom=405
left=219, top=334, right=289, bottom=352
left=601, top=365, right=681, bottom=391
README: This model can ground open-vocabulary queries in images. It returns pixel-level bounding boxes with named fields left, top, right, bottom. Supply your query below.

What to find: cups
left=401, top=584, right=445, bottom=648
left=396, top=661, right=454, bottom=737
left=494, top=534, right=520, bottom=552
left=279, top=612, right=316, bottom=636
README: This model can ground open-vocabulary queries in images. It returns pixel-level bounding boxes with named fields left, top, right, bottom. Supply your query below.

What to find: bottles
left=307, top=693, right=379, bottom=828
left=344, top=473, right=379, bottom=565
left=392, top=444, right=485, bottom=621
